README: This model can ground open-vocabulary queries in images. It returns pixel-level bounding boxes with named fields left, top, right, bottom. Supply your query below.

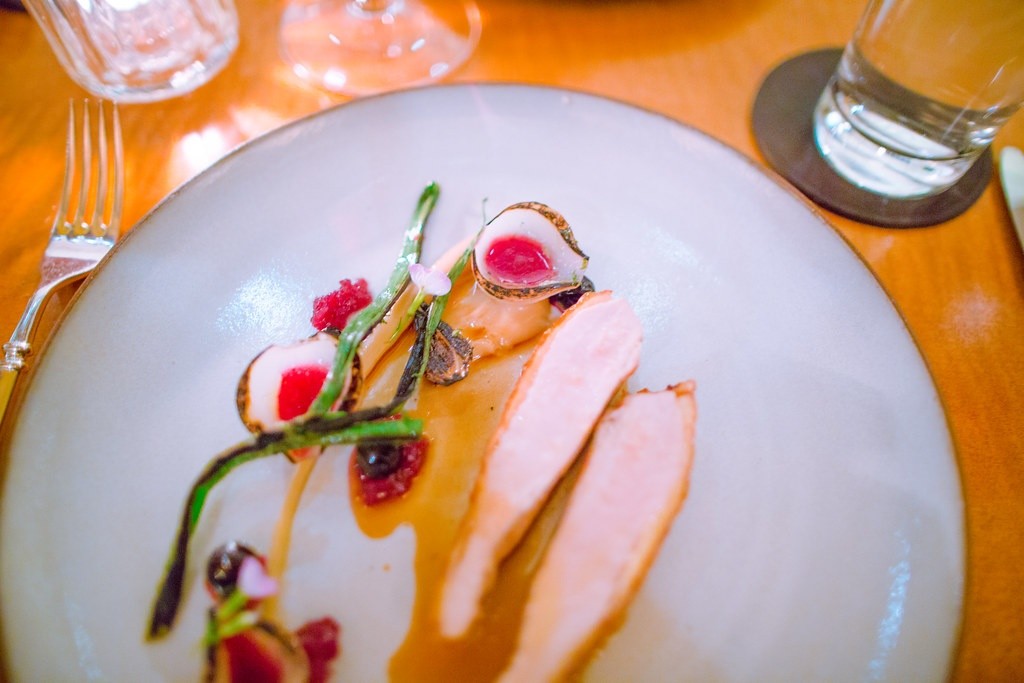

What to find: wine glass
left=276, top=1, right=485, bottom=96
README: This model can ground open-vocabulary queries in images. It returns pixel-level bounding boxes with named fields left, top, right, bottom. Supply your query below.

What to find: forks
left=1, top=98, right=125, bottom=428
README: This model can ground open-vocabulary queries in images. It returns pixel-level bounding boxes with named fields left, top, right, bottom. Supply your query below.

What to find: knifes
left=999, top=144, right=1023, bottom=254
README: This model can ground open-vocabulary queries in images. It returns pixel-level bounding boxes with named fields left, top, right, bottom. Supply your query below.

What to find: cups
left=20, top=1, right=239, bottom=103
left=810, top=0, right=1023, bottom=203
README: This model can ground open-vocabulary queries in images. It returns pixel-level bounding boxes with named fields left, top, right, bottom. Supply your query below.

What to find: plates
left=0, top=82, right=964, bottom=682
